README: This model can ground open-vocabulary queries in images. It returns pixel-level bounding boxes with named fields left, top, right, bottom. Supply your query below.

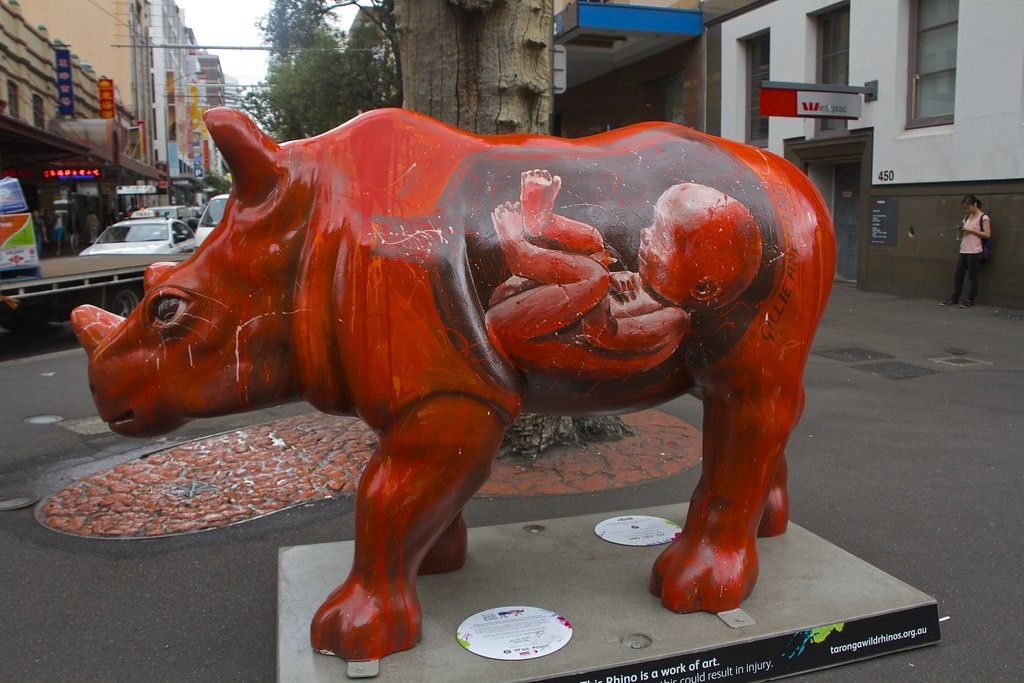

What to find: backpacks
left=962, top=214, right=993, bottom=261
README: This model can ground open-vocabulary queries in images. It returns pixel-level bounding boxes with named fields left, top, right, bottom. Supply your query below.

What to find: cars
left=77, top=193, right=230, bottom=256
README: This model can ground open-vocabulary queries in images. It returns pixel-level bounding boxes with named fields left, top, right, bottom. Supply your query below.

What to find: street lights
left=162, top=71, right=207, bottom=207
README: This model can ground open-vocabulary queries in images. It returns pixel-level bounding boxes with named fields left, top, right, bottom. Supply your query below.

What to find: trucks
left=0, top=176, right=196, bottom=320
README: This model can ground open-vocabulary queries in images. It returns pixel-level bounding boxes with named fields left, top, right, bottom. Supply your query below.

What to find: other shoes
left=940, top=298, right=959, bottom=306
left=960, top=300, right=974, bottom=308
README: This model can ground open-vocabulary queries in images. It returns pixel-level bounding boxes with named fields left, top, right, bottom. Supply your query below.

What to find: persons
left=939, top=194, right=991, bottom=309
left=31, top=207, right=118, bottom=258
